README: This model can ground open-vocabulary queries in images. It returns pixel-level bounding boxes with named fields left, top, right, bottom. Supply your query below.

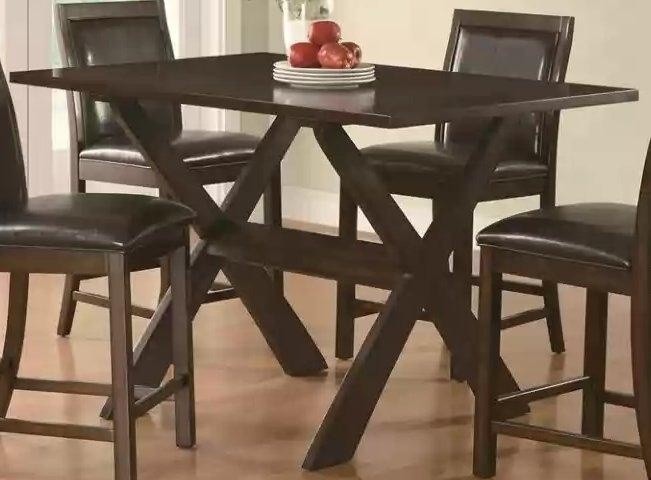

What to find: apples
left=316, top=42, right=354, bottom=69
left=341, top=42, right=362, bottom=69
left=308, top=21, right=341, bottom=46
left=289, top=43, right=318, bottom=67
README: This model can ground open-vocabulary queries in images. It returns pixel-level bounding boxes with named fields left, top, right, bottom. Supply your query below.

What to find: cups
left=277, top=0, right=333, bottom=58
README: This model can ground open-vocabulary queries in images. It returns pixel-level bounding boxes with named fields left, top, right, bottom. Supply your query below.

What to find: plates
left=272, top=61, right=377, bottom=88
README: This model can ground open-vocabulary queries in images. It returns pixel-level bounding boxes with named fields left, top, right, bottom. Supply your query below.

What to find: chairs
left=0, top=57, right=198, bottom=480
left=335, top=8, right=575, bottom=381
left=55, top=4, right=284, bottom=336
left=473, top=139, right=650, bottom=479
